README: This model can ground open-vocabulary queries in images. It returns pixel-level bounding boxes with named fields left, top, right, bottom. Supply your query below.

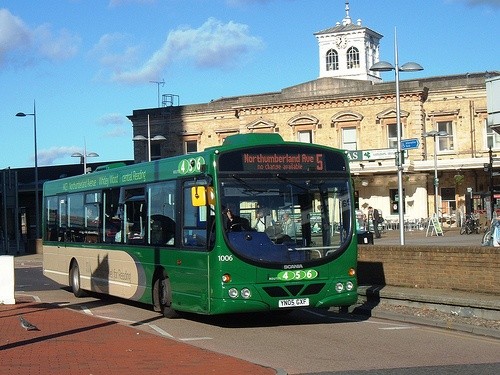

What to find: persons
left=282, top=213, right=297, bottom=238
left=368, top=206, right=381, bottom=239
left=223, top=208, right=243, bottom=232
left=493, top=206, right=500, bottom=248
left=115, top=224, right=130, bottom=243
left=474, top=210, right=480, bottom=233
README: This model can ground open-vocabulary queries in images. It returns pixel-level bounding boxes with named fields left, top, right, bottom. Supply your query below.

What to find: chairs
left=383, top=217, right=429, bottom=231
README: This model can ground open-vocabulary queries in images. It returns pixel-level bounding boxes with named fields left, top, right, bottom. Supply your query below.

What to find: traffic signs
left=400, top=138, right=420, bottom=150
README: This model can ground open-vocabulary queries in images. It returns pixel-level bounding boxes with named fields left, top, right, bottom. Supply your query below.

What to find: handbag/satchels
left=378, top=217, right=384, bottom=223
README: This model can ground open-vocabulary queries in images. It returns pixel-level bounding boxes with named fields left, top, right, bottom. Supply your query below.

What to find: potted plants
left=453, top=174, right=465, bottom=184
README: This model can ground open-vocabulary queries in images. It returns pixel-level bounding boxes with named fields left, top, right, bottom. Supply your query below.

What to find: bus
left=42, top=132, right=360, bottom=320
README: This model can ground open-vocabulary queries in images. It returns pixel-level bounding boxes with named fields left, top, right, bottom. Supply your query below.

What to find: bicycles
left=460, top=212, right=482, bottom=235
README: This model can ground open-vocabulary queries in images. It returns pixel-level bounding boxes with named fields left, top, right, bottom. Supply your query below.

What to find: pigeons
left=19, top=316, right=35, bottom=331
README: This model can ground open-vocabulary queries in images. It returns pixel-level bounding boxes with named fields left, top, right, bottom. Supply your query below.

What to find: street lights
left=132, top=115, right=167, bottom=161
left=15, top=98, right=40, bottom=239
left=368, top=25, right=425, bottom=245
left=71, top=136, right=99, bottom=174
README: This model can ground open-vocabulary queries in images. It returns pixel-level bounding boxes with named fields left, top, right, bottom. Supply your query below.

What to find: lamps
left=360, top=178, right=368, bottom=186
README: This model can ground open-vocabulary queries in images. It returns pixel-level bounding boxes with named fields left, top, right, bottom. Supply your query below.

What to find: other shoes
left=376, top=234, right=381, bottom=238
